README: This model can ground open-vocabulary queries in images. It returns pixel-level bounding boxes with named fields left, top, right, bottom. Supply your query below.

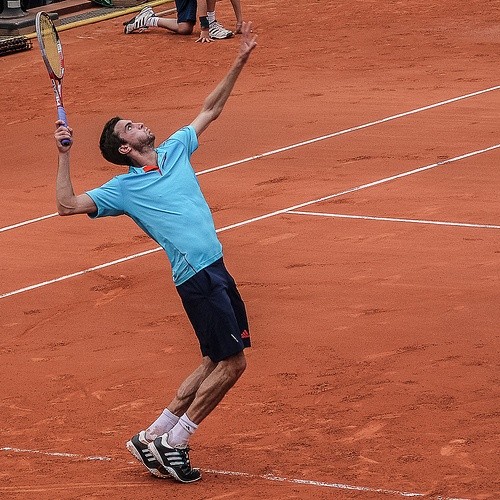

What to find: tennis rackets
left=35, top=9, right=73, bottom=147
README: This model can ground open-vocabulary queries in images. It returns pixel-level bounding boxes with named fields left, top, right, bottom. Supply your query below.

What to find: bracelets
left=236, top=24, right=242, bottom=26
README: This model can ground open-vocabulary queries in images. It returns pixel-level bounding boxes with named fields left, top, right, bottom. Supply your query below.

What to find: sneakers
left=125, top=431, right=202, bottom=483
left=123, top=6, right=155, bottom=34
left=207, top=20, right=235, bottom=39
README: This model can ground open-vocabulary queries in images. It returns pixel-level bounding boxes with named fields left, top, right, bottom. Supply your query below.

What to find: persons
left=125, top=0, right=252, bottom=43
left=54, top=22, right=259, bottom=483
